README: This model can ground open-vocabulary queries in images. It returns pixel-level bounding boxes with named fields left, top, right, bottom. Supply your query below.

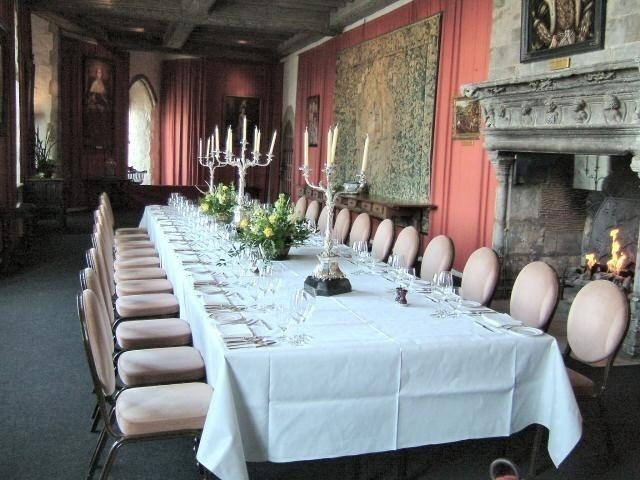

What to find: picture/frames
left=518, top=0, right=608, bottom=63
left=81, top=55, right=118, bottom=148
left=451, top=95, right=482, bottom=141
left=219, top=93, right=263, bottom=149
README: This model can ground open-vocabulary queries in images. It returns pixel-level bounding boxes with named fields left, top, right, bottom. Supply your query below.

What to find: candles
left=197, top=114, right=279, bottom=161
left=302, top=121, right=372, bottom=175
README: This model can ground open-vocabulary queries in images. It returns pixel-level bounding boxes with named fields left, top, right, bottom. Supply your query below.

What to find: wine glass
left=151, top=190, right=317, bottom=350
left=318, top=226, right=547, bottom=338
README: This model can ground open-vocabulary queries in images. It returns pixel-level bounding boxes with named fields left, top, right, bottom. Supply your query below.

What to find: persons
left=307, top=99, right=318, bottom=146
left=234, top=105, right=254, bottom=144
left=90, top=65, right=107, bottom=93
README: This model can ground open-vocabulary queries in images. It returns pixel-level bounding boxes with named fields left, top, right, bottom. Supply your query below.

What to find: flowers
left=198, top=180, right=238, bottom=216
